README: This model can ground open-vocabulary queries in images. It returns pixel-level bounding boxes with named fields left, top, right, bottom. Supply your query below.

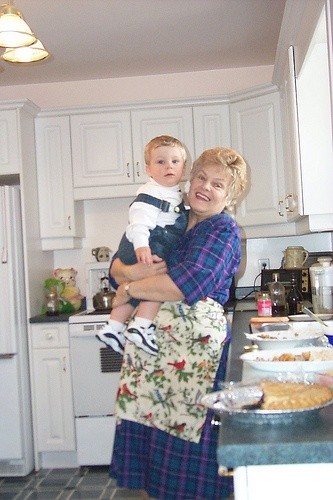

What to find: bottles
left=46, top=293, right=60, bottom=316
left=307, top=256, right=333, bottom=316
left=286, top=273, right=305, bottom=315
left=258, top=293, right=273, bottom=316
left=269, top=272, right=287, bottom=317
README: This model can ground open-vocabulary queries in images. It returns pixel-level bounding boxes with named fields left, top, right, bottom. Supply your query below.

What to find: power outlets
left=259, top=259, right=270, bottom=274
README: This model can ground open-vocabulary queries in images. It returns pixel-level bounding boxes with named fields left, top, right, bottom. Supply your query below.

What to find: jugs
left=92, top=276, right=116, bottom=311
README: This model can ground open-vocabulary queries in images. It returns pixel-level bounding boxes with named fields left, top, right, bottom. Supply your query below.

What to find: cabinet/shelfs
left=279, top=46, right=303, bottom=223
left=34, top=115, right=74, bottom=237
left=70, top=106, right=194, bottom=187
left=31, top=322, right=76, bottom=451
left=230, top=93, right=288, bottom=227
left=0, top=109, right=20, bottom=174
left=192, top=105, right=230, bottom=162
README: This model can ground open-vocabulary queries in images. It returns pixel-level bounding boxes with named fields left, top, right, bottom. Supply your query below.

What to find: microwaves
left=261, top=268, right=312, bottom=301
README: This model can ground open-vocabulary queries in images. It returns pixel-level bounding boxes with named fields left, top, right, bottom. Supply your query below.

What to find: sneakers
left=95, top=320, right=126, bottom=357
left=123, top=317, right=160, bottom=357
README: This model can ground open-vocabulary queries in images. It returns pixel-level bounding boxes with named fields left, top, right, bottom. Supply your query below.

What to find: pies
left=259, top=381, right=332, bottom=409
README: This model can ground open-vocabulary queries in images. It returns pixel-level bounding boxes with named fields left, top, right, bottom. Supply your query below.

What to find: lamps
left=0, top=0, right=37, bottom=48
left=2, top=39, right=48, bottom=64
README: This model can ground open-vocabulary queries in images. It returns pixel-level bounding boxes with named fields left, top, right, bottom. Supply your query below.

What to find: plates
left=288, top=313, right=333, bottom=321
left=200, top=381, right=333, bottom=425
left=245, top=330, right=324, bottom=349
left=239, top=346, right=333, bottom=373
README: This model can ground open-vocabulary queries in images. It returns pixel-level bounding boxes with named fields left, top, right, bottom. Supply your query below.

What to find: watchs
left=125, top=279, right=136, bottom=300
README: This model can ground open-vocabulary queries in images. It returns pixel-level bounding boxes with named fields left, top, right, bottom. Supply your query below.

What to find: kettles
left=282, top=246, right=309, bottom=270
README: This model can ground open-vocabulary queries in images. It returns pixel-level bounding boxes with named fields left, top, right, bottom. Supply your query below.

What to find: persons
left=94, top=135, right=192, bottom=358
left=106, top=146, right=249, bottom=500
